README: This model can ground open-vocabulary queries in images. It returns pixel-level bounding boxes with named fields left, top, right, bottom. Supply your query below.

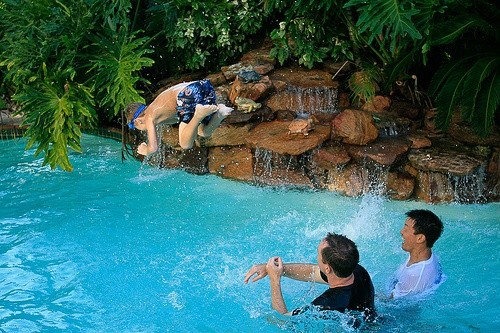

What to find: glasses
left=127, top=104, right=145, bottom=130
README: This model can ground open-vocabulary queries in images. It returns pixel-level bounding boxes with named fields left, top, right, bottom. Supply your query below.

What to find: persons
left=126, top=80, right=233, bottom=156
left=245, top=233, right=375, bottom=316
left=388, top=209, right=444, bottom=299
